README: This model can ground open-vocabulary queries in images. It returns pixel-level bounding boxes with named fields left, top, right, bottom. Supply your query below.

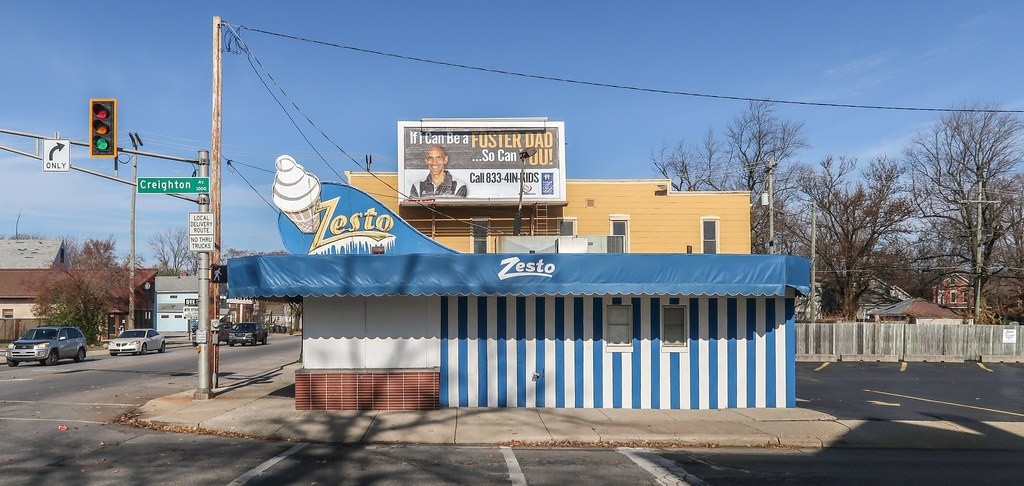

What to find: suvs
left=193, top=322, right=233, bottom=348
left=6, top=325, right=87, bottom=366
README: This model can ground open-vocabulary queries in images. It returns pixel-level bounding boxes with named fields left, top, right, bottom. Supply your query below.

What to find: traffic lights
left=210, top=265, right=228, bottom=283
left=91, top=98, right=118, bottom=158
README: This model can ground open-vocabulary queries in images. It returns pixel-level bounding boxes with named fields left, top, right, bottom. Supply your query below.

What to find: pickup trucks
left=228, top=321, right=268, bottom=347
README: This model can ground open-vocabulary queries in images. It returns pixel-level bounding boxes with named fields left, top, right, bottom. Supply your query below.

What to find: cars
left=107, top=328, right=166, bottom=356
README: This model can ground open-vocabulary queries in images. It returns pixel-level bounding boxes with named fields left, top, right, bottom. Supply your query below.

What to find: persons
left=409, top=144, right=468, bottom=197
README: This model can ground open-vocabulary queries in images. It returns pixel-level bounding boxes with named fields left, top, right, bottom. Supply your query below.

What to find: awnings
left=227, top=253, right=814, bottom=298
